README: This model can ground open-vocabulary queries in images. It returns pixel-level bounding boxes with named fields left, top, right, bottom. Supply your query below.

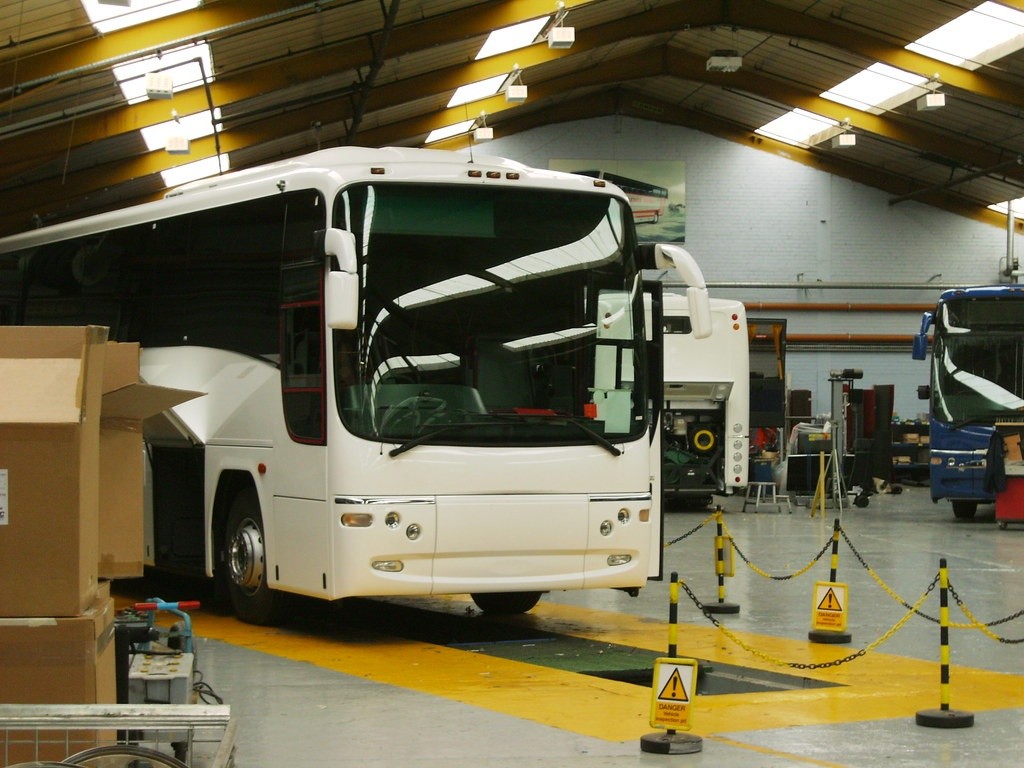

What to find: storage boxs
left=892, top=416, right=899, bottom=422
left=898, top=456, right=911, bottom=463
left=898, top=463, right=910, bottom=465
left=920, top=436, right=930, bottom=443
left=922, top=420, right=926, bottom=424
left=918, top=448, right=929, bottom=463
left=893, top=461, right=898, bottom=465
left=903, top=433, right=919, bottom=444
left=892, top=457, right=898, bottom=461
left=0, top=326, right=209, bottom=768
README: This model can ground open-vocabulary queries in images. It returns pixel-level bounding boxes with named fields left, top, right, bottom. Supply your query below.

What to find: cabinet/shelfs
left=890, top=425, right=930, bottom=488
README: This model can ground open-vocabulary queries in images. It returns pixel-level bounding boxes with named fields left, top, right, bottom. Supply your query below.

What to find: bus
left=912, top=284, right=1024, bottom=522
left=593, top=288, right=752, bottom=509
left=0, top=144, right=713, bottom=626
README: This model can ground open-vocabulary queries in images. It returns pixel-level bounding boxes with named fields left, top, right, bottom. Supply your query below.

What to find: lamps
left=166, top=109, right=191, bottom=155
left=547, top=2, right=576, bottom=49
left=915, top=73, right=947, bottom=112
left=832, top=117, right=857, bottom=148
left=505, top=63, right=528, bottom=103
left=474, top=110, right=494, bottom=142
left=145, top=48, right=176, bottom=100
